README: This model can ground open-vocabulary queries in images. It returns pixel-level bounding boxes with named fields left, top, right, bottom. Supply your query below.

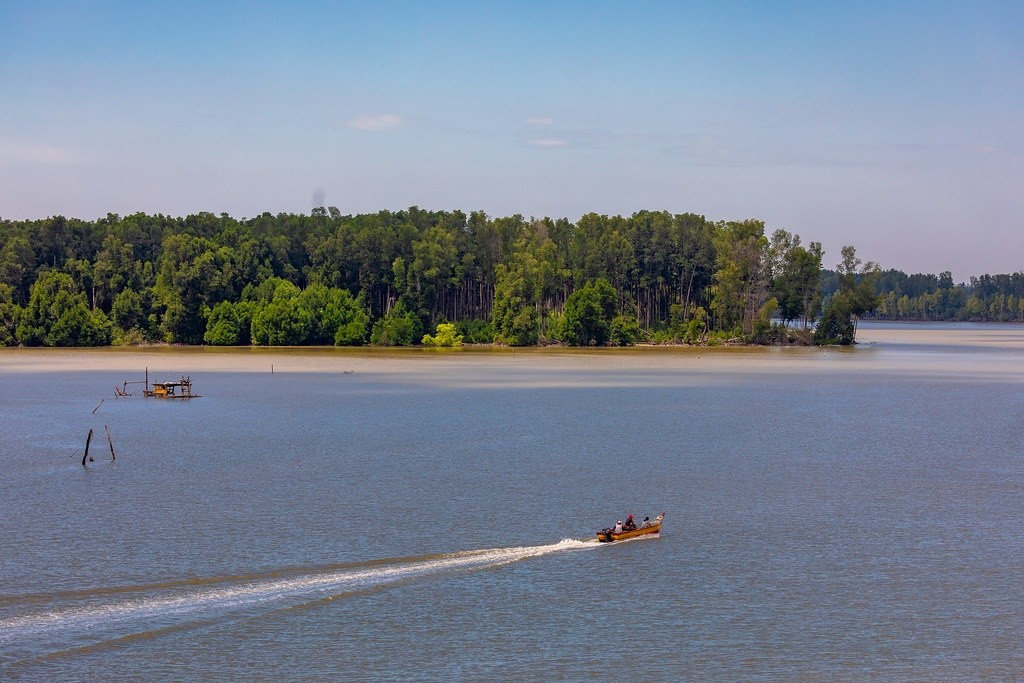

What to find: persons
left=612, top=521, right=625, bottom=533
left=640, top=517, right=651, bottom=529
left=625, top=514, right=636, bottom=530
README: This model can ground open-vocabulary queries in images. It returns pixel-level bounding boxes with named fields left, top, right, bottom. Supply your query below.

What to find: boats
left=596, top=511, right=665, bottom=542
